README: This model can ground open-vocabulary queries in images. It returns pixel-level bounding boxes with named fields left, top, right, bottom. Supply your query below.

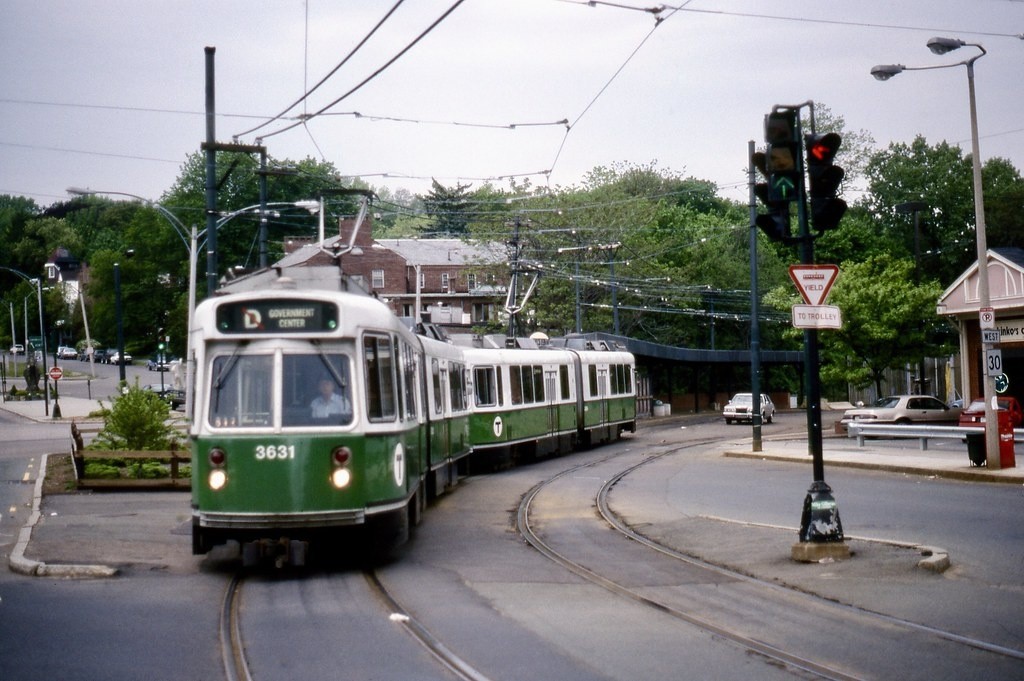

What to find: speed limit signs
left=986, top=349, right=1002, bottom=378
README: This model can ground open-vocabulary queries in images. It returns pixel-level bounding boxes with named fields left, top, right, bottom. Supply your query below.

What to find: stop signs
left=48, top=366, right=63, bottom=381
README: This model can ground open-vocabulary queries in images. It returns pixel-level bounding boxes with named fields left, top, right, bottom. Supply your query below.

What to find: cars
left=56, top=345, right=133, bottom=365
left=839, top=395, right=964, bottom=441
left=9, top=344, right=24, bottom=355
left=959, top=394, right=1024, bottom=428
left=722, top=392, right=776, bottom=425
left=148, top=358, right=171, bottom=372
left=140, top=383, right=186, bottom=410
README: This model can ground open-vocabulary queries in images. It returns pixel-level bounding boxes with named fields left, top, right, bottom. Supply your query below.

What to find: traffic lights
left=751, top=150, right=791, bottom=242
left=802, top=132, right=848, bottom=231
left=764, top=109, right=803, bottom=203
left=157, top=326, right=166, bottom=352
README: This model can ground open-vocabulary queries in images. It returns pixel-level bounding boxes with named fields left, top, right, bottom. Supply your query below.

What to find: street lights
left=47, top=275, right=96, bottom=376
left=369, top=244, right=422, bottom=324
left=29, top=278, right=51, bottom=417
left=65, top=183, right=322, bottom=421
left=25, top=287, right=50, bottom=362
left=869, top=35, right=1001, bottom=471
left=892, top=199, right=927, bottom=394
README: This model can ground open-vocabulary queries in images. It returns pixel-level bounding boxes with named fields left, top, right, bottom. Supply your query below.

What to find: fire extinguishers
left=996, top=409, right=1015, bottom=468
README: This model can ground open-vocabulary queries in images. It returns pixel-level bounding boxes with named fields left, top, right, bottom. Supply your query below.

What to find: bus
left=184, top=187, right=637, bottom=582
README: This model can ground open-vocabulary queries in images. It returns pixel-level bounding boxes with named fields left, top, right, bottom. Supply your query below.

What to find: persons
left=310, top=372, right=353, bottom=419
